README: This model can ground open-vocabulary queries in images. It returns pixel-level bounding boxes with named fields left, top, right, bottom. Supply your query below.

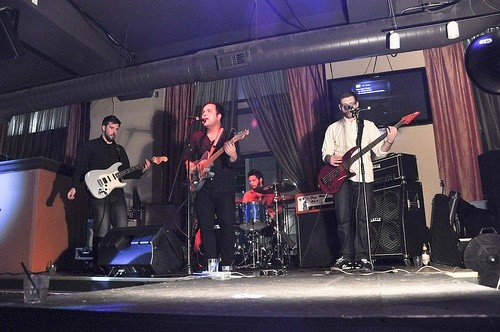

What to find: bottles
left=421, top=244, right=430, bottom=266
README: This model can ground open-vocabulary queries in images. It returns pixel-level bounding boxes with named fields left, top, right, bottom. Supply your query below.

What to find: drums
left=233, top=201, right=266, bottom=230
left=218, top=225, right=251, bottom=268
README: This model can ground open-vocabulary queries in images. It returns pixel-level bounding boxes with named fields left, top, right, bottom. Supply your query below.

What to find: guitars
left=317, top=111, right=421, bottom=194
left=189, top=128, right=249, bottom=191
left=84, top=156, right=169, bottom=198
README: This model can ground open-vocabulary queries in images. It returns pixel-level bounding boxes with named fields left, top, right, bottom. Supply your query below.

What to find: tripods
left=235, top=195, right=298, bottom=269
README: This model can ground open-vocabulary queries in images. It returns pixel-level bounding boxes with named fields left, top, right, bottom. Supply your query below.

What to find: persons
left=321, top=90, right=398, bottom=270
left=182, top=101, right=239, bottom=271
left=243, top=169, right=283, bottom=217
left=68, top=115, right=152, bottom=276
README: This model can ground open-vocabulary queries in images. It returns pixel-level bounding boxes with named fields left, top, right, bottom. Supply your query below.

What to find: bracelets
left=231, top=152, right=237, bottom=158
left=387, top=140, right=394, bottom=144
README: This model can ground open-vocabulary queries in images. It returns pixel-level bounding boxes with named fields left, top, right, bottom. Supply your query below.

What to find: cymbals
left=271, top=195, right=296, bottom=204
left=255, top=183, right=296, bottom=194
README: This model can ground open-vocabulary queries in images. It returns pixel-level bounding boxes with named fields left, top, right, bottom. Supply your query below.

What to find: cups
left=24, top=275, right=50, bottom=305
left=221, top=265, right=233, bottom=272
left=207, top=259, right=219, bottom=273
left=413, top=256, right=423, bottom=267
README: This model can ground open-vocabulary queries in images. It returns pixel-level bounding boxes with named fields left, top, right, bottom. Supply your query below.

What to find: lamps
left=446, top=20, right=459, bottom=39
left=389, top=30, right=400, bottom=50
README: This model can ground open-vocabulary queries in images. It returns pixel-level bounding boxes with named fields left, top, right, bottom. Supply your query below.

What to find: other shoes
left=342, top=259, right=373, bottom=272
left=330, top=256, right=354, bottom=272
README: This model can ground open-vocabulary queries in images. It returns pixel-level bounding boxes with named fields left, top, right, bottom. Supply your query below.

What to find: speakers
left=368, top=181, right=427, bottom=262
left=99, top=226, right=188, bottom=276
left=478, top=150, right=500, bottom=232
left=432, top=226, right=473, bottom=267
left=141, top=203, right=188, bottom=247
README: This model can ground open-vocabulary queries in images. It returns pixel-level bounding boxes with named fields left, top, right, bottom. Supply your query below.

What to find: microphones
left=188, top=117, right=206, bottom=122
left=347, top=105, right=354, bottom=110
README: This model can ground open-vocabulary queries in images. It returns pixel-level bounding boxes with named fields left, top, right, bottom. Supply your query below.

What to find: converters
left=297, top=211, right=342, bottom=270
left=209, top=272, right=231, bottom=280
